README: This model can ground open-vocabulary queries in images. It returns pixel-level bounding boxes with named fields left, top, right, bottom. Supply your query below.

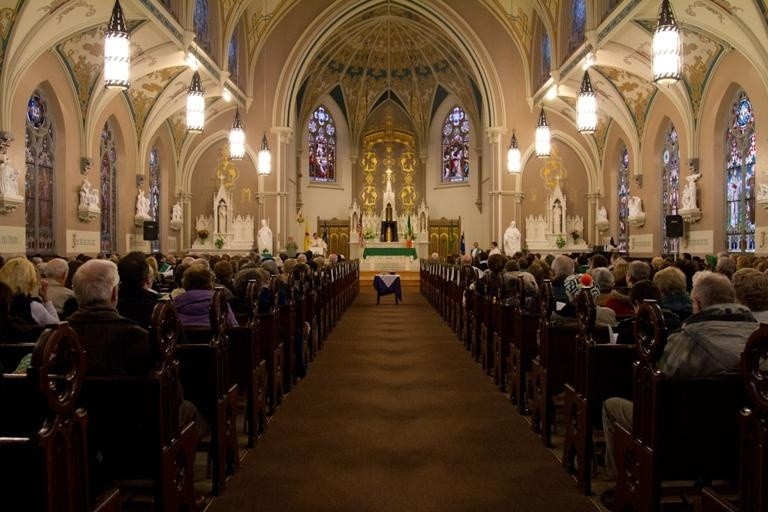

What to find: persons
left=171, top=200, right=185, bottom=222
left=1, top=247, right=346, bottom=511
left=681, top=169, right=701, bottom=210
left=627, top=196, right=643, bottom=216
left=553, top=203, right=561, bottom=233
left=284, top=230, right=329, bottom=259
left=0, top=158, right=21, bottom=196
left=256, top=219, right=274, bottom=256
left=425, top=239, right=768, bottom=511
left=502, top=220, right=522, bottom=256
left=134, top=187, right=150, bottom=215
left=78, top=178, right=100, bottom=209
left=218, top=202, right=227, bottom=233
left=596, top=205, right=608, bottom=221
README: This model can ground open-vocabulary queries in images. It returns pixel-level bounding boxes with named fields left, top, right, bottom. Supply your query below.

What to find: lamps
left=576, top=42, right=598, bottom=135
left=186, top=1, right=205, bottom=134
left=534, top=63, right=552, bottom=158
left=229, top=24, right=247, bottom=160
left=257, top=133, right=272, bottom=175
left=506, top=131, right=523, bottom=174
left=103, top=0, right=131, bottom=91
left=650, top=0, right=684, bottom=86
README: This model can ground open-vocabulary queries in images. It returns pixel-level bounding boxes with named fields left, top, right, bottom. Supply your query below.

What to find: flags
left=356, top=225, right=364, bottom=246
left=461, top=236, right=465, bottom=252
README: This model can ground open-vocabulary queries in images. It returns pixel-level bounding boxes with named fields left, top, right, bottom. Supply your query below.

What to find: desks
left=375, top=275, right=400, bottom=305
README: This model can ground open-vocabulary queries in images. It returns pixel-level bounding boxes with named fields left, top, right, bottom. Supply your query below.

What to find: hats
left=705, top=254, right=717, bottom=268
left=564, top=273, right=600, bottom=306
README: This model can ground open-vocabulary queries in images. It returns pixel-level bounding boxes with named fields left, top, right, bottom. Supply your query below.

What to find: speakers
left=144, top=221, right=159, bottom=240
left=666, top=215, right=683, bottom=236
